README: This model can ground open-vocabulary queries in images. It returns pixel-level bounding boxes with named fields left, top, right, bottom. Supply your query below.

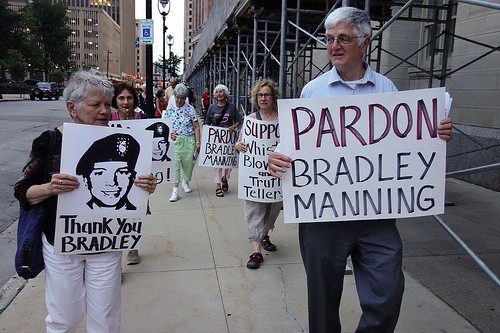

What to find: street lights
left=166, top=33, right=175, bottom=78
left=157, top=0, right=171, bottom=90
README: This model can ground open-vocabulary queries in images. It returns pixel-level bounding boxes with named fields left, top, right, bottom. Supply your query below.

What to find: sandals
left=215, top=187, right=224, bottom=198
left=222, top=175, right=229, bottom=192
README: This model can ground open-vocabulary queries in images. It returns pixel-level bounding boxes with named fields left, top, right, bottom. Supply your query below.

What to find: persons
left=108, top=84, right=149, bottom=265
left=163, top=83, right=201, bottom=201
left=135, top=78, right=198, bottom=122
left=200, top=87, right=210, bottom=125
left=235, top=77, right=284, bottom=268
left=270, top=7, right=453, bottom=333
left=204, top=85, right=241, bottom=196
left=15, top=70, right=157, bottom=333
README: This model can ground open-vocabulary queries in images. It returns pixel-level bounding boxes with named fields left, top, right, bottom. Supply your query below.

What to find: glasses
left=325, top=35, right=361, bottom=44
left=257, top=92, right=274, bottom=99
left=69, top=78, right=114, bottom=95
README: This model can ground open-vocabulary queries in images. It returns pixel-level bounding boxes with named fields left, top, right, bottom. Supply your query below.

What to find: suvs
left=29, top=82, right=60, bottom=101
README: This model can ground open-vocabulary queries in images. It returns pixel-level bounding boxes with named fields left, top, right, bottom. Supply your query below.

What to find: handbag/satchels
left=13, top=206, right=47, bottom=280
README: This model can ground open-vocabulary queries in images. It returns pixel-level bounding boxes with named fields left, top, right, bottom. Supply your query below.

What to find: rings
left=60, top=179, right=62, bottom=184
left=62, top=185, right=63, bottom=189
left=150, top=180, right=152, bottom=184
left=149, top=185, right=151, bottom=188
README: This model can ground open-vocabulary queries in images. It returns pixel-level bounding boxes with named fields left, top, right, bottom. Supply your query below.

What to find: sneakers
left=126, top=249, right=140, bottom=265
left=181, top=181, right=192, bottom=193
left=169, top=191, right=180, bottom=201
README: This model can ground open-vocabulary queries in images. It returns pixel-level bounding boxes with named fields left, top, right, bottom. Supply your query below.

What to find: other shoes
left=246, top=252, right=264, bottom=269
left=343, top=264, right=352, bottom=275
left=260, top=234, right=277, bottom=252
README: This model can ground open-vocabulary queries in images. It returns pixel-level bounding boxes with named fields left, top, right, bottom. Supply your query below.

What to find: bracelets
left=196, top=141, right=200, bottom=143
